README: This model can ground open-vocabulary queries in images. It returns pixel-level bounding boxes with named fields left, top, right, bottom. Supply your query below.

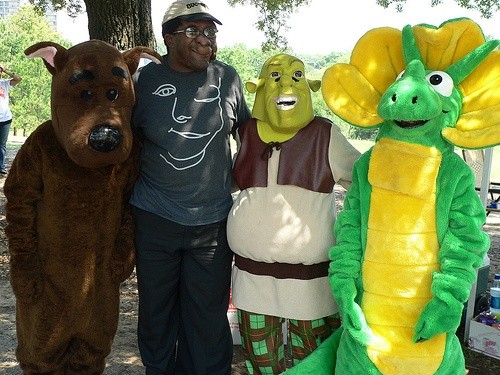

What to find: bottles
left=490, top=274, right=500, bottom=313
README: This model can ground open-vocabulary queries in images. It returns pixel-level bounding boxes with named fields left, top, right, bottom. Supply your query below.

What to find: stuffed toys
left=5, top=39, right=164, bottom=375
left=273, top=16, right=500, bottom=375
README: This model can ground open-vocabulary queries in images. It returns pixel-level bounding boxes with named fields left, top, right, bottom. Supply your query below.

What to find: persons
left=226, top=53, right=364, bottom=375
left=0, top=64, right=22, bottom=174
left=125, top=0, right=253, bottom=375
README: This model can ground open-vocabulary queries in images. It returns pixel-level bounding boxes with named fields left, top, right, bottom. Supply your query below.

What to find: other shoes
left=0, top=170, right=8, bottom=174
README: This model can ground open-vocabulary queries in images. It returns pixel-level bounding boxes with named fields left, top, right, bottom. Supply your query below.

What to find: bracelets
left=10, top=73, right=14, bottom=77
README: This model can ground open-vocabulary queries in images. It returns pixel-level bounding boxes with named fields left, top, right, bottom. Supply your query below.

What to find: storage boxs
left=468, top=311, right=500, bottom=360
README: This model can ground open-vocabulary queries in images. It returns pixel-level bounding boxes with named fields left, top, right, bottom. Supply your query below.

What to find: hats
left=162, top=0, right=222, bottom=34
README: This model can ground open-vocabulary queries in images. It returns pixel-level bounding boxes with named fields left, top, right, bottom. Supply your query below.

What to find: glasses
left=169, top=25, right=217, bottom=38
